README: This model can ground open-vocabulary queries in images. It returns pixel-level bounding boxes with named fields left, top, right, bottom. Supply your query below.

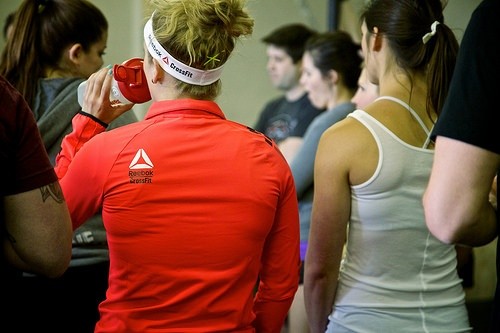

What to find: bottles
left=76, top=57, right=152, bottom=109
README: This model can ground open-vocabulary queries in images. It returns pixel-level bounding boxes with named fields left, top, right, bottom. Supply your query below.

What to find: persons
left=276, top=31, right=365, bottom=333
left=0, top=1, right=139, bottom=332
left=303, top=0, right=461, bottom=333
left=55, top=0, right=301, bottom=333
left=256, top=22, right=327, bottom=165
left=1, top=74, right=74, bottom=333
left=350, top=51, right=381, bottom=110
left=423, top=0, right=499, bottom=302
